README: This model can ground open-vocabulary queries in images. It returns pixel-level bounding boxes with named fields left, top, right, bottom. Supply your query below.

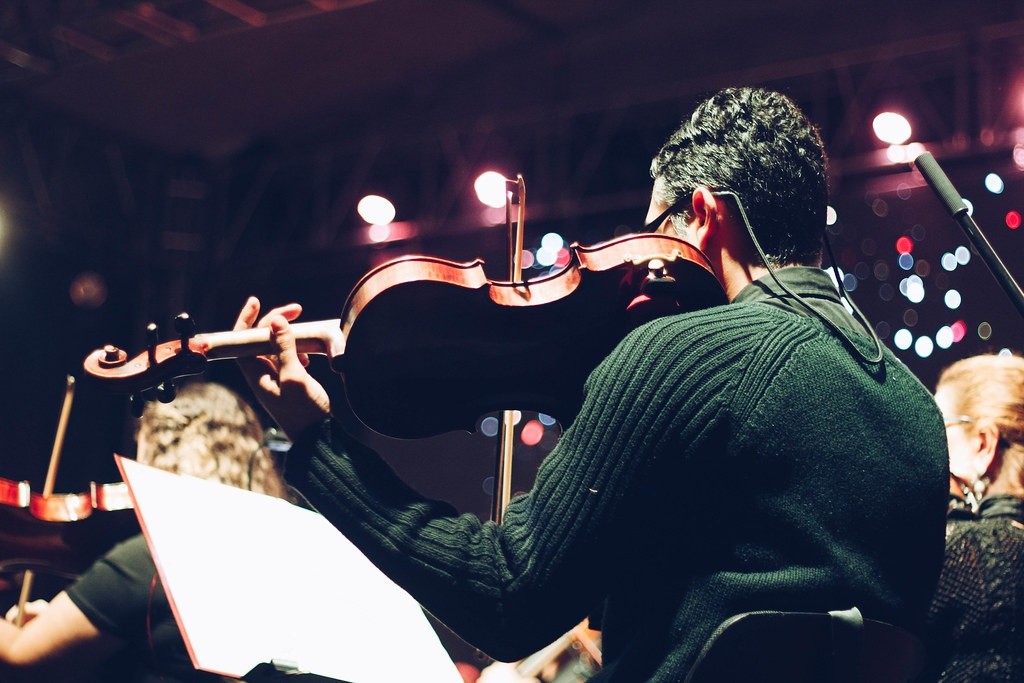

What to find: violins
left=0, top=474, right=144, bottom=577
left=76, top=230, right=733, bottom=424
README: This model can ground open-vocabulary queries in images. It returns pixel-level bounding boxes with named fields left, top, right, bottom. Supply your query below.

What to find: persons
left=233, top=86, right=951, bottom=683
left=933, top=355, right=1024, bottom=683
left=0, top=381, right=283, bottom=683
